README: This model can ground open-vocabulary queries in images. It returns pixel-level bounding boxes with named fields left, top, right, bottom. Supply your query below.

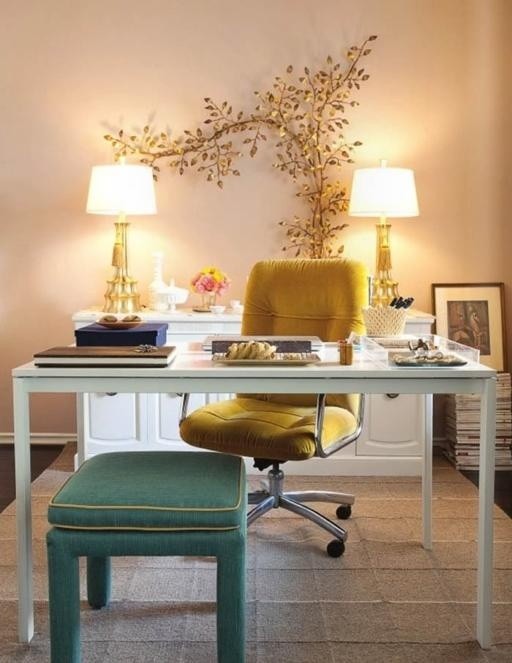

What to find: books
left=34, top=344, right=176, bottom=368
left=441, top=370, right=512, bottom=469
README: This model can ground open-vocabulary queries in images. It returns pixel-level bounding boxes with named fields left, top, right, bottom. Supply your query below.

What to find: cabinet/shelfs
left=74, top=320, right=433, bottom=476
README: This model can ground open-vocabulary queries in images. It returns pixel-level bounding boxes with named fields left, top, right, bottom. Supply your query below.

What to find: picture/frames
left=432, top=282, right=509, bottom=373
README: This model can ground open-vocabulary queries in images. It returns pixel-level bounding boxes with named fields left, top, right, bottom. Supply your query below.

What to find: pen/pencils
left=389, top=297, right=414, bottom=309
left=347, top=332, right=356, bottom=344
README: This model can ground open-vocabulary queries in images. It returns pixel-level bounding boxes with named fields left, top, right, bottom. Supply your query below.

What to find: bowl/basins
left=209, top=305, right=226, bottom=313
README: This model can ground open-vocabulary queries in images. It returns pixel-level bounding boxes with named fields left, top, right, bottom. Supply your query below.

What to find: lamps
left=85, top=164, right=157, bottom=312
left=348, top=166, right=422, bottom=306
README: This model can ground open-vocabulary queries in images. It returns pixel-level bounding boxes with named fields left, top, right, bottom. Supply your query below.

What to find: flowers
left=190, top=267, right=231, bottom=296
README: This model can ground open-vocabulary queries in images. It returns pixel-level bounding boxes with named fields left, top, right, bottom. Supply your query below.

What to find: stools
left=47, top=451, right=247, bottom=663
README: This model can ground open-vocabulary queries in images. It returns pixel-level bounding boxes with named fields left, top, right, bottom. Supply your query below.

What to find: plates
left=212, top=350, right=321, bottom=366
left=96, top=319, right=142, bottom=330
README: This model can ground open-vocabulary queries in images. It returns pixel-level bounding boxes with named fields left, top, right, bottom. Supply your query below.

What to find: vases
left=201, top=293, right=216, bottom=306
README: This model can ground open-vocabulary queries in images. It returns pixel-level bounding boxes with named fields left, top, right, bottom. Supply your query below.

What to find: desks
left=12, top=346, right=497, bottom=645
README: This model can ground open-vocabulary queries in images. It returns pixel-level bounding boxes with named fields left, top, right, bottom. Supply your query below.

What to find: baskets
left=360, top=305, right=408, bottom=338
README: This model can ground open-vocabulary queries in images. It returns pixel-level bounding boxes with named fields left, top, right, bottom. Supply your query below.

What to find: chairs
left=179, top=259, right=364, bottom=559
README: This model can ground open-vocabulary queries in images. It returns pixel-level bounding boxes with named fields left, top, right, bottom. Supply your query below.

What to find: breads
left=225, top=341, right=275, bottom=359
